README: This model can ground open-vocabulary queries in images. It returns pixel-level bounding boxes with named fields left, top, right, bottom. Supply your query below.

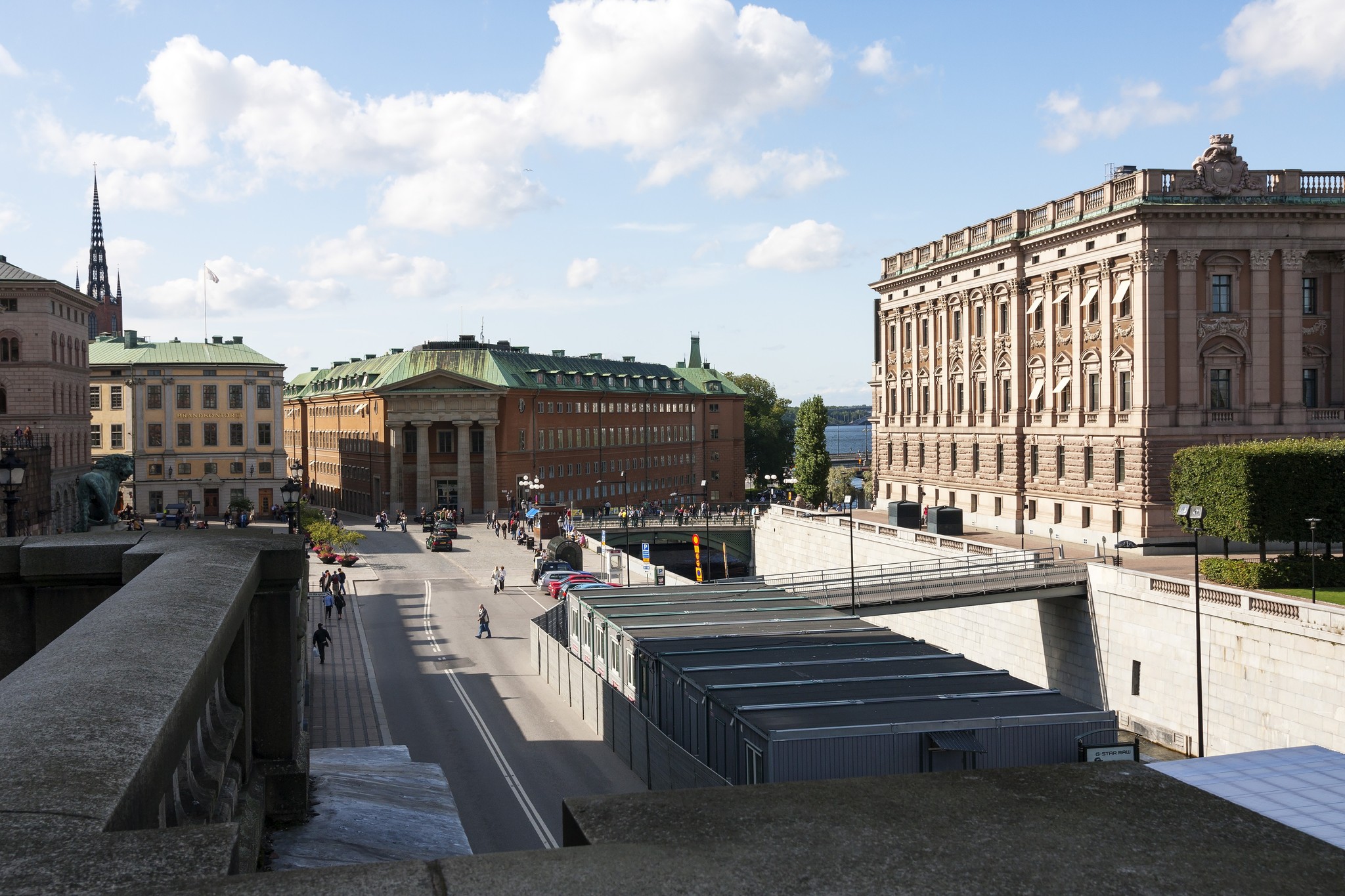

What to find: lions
left=76, top=453, right=135, bottom=530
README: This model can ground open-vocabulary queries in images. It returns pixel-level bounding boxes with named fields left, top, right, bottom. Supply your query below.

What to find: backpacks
left=332, top=574, right=339, bottom=584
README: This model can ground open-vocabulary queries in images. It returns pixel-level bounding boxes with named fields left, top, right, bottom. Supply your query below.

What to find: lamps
left=38, top=500, right=60, bottom=517
left=250, top=464, right=254, bottom=476
left=307, top=476, right=314, bottom=481
left=69, top=474, right=80, bottom=486
left=169, top=466, right=173, bottom=478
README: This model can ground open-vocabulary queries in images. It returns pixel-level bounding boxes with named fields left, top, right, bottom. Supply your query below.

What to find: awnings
left=525, top=508, right=536, bottom=518
left=529, top=510, right=539, bottom=519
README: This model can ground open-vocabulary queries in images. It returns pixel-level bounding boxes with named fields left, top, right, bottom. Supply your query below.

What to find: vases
left=319, top=558, right=336, bottom=564
left=337, top=560, right=358, bottom=566
left=314, top=550, right=332, bottom=555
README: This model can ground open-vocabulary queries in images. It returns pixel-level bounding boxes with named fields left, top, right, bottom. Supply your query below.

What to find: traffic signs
left=308, top=592, right=329, bottom=597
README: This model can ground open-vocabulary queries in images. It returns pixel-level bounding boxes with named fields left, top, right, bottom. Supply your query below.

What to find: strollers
left=117, top=509, right=128, bottom=520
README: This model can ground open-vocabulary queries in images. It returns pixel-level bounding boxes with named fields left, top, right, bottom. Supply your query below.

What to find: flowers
left=310, top=540, right=328, bottom=544
left=312, top=544, right=333, bottom=551
left=317, top=551, right=338, bottom=558
left=305, top=533, right=310, bottom=537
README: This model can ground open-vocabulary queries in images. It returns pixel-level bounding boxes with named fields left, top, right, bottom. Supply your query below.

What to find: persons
left=118, top=493, right=842, bottom=664
left=25, top=426, right=33, bottom=449
left=14, top=425, right=24, bottom=450
left=923, top=505, right=929, bottom=525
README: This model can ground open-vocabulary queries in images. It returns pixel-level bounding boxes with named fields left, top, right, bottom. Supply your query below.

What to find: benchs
left=522, top=535, right=529, bottom=546
left=532, top=546, right=539, bottom=553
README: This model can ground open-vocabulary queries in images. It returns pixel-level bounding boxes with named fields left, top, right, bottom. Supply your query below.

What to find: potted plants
left=229, top=497, right=255, bottom=527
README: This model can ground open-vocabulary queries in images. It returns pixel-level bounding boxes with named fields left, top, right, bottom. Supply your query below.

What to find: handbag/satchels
left=386, top=519, right=390, bottom=524
left=313, top=646, right=320, bottom=657
left=343, top=602, right=345, bottom=607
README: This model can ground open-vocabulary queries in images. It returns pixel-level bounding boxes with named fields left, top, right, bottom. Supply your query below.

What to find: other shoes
left=498, top=592, right=500, bottom=593
left=500, top=589, right=503, bottom=591
left=338, top=617, right=342, bottom=620
left=475, top=636, right=481, bottom=639
left=325, top=618, right=327, bottom=619
left=486, top=635, right=491, bottom=638
left=420, top=523, right=422, bottom=525
left=403, top=531, right=407, bottom=533
left=343, top=592, right=346, bottom=595
left=387, top=526, right=389, bottom=529
left=494, top=593, right=496, bottom=595
left=319, top=660, right=324, bottom=664
left=401, top=529, right=404, bottom=530
left=329, top=618, right=331, bottom=619
left=377, top=528, right=380, bottom=529
left=424, top=524, right=427, bottom=525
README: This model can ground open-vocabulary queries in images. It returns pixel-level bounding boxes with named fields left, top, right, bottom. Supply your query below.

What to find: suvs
left=414, top=510, right=449, bottom=524
left=757, top=489, right=786, bottom=500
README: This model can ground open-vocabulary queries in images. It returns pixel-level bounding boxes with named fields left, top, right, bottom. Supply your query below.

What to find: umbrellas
left=565, top=519, right=570, bottom=539
left=562, top=517, right=567, bottom=538
left=570, top=520, right=574, bottom=533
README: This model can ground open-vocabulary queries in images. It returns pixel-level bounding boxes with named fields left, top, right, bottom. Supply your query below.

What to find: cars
left=571, top=583, right=614, bottom=589
left=426, top=532, right=453, bottom=551
left=531, top=559, right=623, bottom=604
left=423, top=520, right=458, bottom=538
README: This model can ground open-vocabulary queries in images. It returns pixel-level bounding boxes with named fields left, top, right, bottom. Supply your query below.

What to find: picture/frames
left=335, top=555, right=360, bottom=561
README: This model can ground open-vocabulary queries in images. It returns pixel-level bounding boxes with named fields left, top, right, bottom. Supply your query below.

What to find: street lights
left=506, top=492, right=512, bottom=534
left=518, top=475, right=545, bottom=551
left=279, top=475, right=302, bottom=534
left=289, top=459, right=305, bottom=534
left=1114, top=502, right=1205, bottom=758
left=916, top=479, right=925, bottom=533
left=841, top=472, right=848, bottom=515
left=305, top=536, right=312, bottom=599
left=596, top=471, right=630, bottom=587
left=830, top=422, right=839, bottom=456
left=1112, top=499, right=1124, bottom=566
left=1305, top=517, right=1316, bottom=606
left=862, top=422, right=871, bottom=466
left=749, top=467, right=798, bottom=508
left=844, top=495, right=856, bottom=616
left=669, top=480, right=711, bottom=584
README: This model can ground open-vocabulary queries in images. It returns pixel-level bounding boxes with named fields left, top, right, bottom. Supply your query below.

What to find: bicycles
left=130, top=510, right=143, bottom=519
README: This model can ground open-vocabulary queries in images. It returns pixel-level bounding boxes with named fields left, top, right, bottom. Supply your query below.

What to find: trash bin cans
left=526, top=537, right=535, bottom=549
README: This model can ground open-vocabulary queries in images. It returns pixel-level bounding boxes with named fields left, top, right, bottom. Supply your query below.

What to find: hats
left=318, top=623, right=323, bottom=628
